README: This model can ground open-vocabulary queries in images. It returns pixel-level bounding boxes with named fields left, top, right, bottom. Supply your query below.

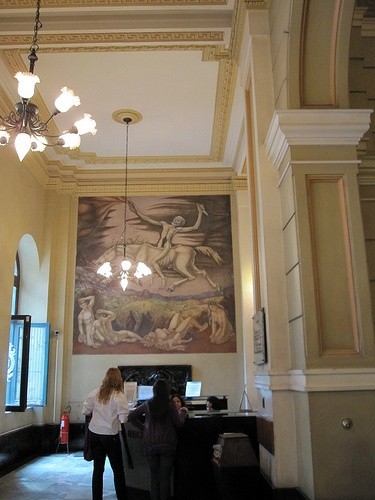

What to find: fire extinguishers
left=57, top=406, right=73, bottom=446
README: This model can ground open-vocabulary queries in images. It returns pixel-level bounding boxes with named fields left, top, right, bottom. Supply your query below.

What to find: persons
left=82, top=367, right=129, bottom=500
left=206, top=396, right=222, bottom=410
left=128, top=379, right=188, bottom=500
left=170, top=394, right=185, bottom=409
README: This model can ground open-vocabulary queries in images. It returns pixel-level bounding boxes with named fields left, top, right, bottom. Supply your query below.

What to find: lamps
left=96, top=109, right=153, bottom=294
left=0, top=2, right=97, bottom=163
